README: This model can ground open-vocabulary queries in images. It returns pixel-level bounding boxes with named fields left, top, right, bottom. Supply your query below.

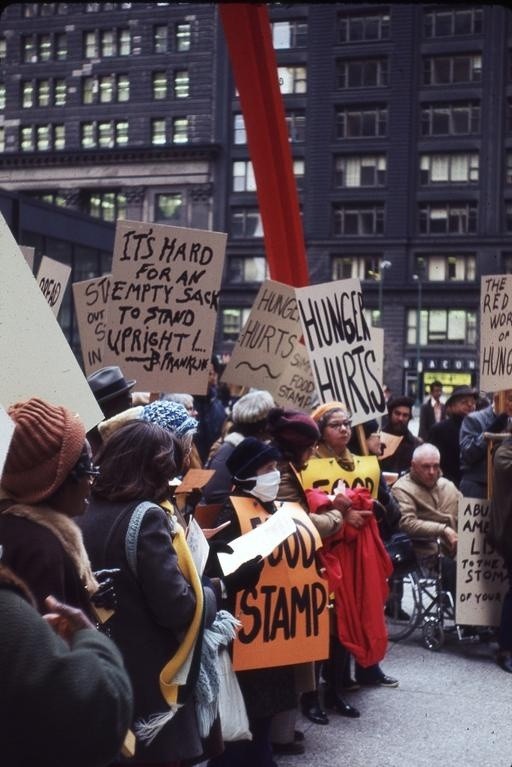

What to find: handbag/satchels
left=130, top=694, right=204, bottom=765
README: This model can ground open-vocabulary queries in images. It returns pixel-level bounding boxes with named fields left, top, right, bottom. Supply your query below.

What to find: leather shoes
left=496, top=653, right=512, bottom=674
left=323, top=690, right=361, bottom=718
left=301, top=692, right=329, bottom=725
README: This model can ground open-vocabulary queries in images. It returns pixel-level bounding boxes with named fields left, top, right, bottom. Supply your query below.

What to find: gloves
left=90, top=565, right=120, bottom=613
left=486, top=412, right=509, bottom=435
left=200, top=535, right=267, bottom=598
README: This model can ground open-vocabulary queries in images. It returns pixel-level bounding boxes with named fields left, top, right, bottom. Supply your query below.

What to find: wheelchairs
left=382, top=525, right=492, bottom=651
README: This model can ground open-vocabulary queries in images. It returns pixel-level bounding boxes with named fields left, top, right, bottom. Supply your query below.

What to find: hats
left=225, top=400, right=351, bottom=486
left=0, top=395, right=86, bottom=506
left=387, top=396, right=415, bottom=409
left=444, top=384, right=479, bottom=408
left=86, top=366, right=198, bottom=447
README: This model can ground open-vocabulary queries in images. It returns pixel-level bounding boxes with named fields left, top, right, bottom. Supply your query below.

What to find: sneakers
left=272, top=728, right=305, bottom=755
left=325, top=677, right=361, bottom=691
left=357, top=674, right=398, bottom=688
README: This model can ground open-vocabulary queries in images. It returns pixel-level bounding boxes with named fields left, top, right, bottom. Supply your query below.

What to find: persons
left=3, top=358, right=511, bottom=767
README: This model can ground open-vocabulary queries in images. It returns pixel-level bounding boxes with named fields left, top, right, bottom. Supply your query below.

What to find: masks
left=251, top=471, right=281, bottom=503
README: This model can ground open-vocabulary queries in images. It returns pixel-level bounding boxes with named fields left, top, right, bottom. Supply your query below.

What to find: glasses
left=78, top=465, right=102, bottom=487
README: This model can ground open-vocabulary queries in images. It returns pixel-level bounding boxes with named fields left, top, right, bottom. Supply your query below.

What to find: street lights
left=408, top=273, right=426, bottom=414
left=374, top=256, right=394, bottom=333
